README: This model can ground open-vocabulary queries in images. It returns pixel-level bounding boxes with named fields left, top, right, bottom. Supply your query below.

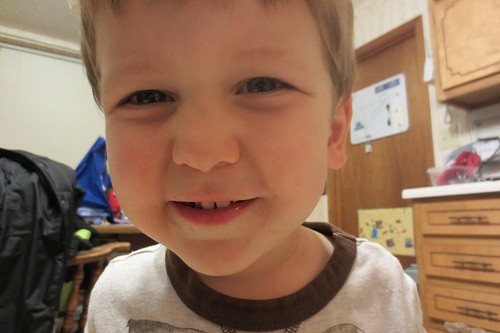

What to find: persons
left=79, top=0, right=422, bottom=333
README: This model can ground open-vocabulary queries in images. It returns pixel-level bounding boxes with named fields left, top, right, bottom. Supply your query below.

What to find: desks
left=84, top=226, right=143, bottom=250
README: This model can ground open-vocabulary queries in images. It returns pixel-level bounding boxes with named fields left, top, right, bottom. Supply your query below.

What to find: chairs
left=58, top=241, right=132, bottom=333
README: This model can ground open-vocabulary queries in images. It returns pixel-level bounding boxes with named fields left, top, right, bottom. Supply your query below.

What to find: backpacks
left=0, top=148, right=97, bottom=333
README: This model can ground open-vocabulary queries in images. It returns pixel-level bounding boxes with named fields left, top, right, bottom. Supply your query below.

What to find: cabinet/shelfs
left=400, top=175, right=500, bottom=333
left=427, top=0, right=500, bottom=104
